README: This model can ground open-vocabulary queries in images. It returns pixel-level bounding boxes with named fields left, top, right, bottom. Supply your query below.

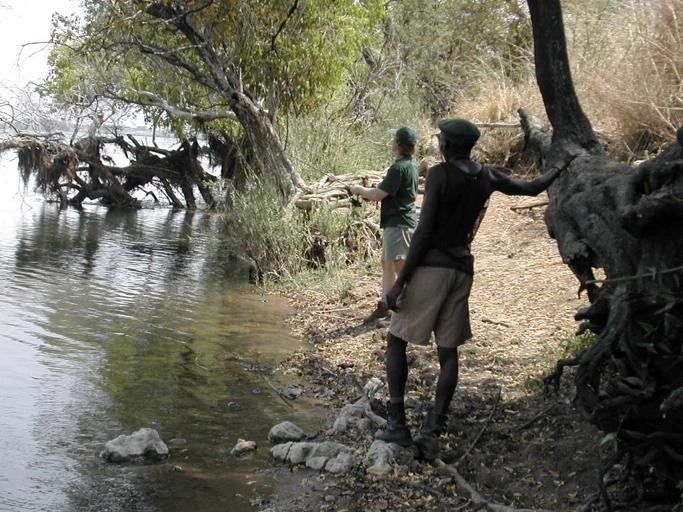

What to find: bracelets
left=552, top=166, right=561, bottom=173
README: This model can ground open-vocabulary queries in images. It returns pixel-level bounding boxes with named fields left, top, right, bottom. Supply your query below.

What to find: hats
left=389, top=126, right=416, bottom=142
left=434, top=117, right=483, bottom=142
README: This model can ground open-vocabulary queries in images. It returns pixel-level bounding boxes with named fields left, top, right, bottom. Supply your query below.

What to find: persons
left=374, top=117, right=581, bottom=460
left=349, top=126, right=419, bottom=321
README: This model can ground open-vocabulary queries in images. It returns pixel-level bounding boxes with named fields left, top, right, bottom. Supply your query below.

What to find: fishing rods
left=284, top=151, right=362, bottom=207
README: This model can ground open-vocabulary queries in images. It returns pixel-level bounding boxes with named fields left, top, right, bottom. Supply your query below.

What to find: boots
left=374, top=401, right=411, bottom=446
left=412, top=412, right=447, bottom=460
